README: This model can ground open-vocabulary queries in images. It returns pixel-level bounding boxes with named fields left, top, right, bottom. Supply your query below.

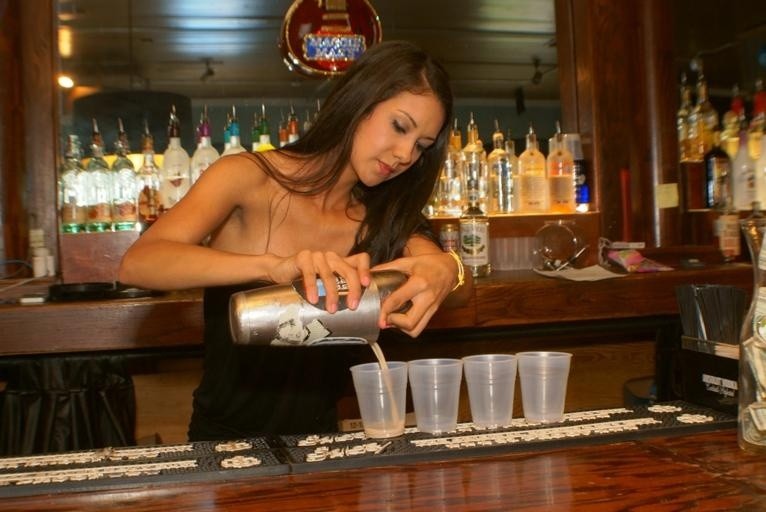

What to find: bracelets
left=447, top=249, right=466, bottom=293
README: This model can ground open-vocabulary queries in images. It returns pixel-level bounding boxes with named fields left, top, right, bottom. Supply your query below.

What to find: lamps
left=193, top=58, right=219, bottom=92
left=530, top=55, right=546, bottom=88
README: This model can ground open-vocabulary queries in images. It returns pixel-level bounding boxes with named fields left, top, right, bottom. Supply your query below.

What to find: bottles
left=60, top=97, right=319, bottom=234
left=425, top=107, right=591, bottom=280
left=737, top=215, right=765, bottom=457
left=675, top=67, right=766, bottom=265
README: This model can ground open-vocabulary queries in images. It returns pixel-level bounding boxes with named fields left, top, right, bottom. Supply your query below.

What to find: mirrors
left=47, top=1, right=577, bottom=233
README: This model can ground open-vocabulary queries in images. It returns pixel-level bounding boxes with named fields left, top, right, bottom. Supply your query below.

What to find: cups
left=514, top=348, right=575, bottom=425
left=227, top=269, right=384, bottom=352
left=407, top=356, right=465, bottom=436
left=463, top=351, right=520, bottom=430
left=347, top=361, right=409, bottom=442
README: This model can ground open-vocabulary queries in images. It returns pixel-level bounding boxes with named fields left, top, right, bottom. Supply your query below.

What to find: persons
left=114, top=38, right=474, bottom=442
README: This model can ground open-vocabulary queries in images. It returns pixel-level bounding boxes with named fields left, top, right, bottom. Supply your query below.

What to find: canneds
left=439, top=223, right=460, bottom=252
left=457, top=219, right=493, bottom=278
